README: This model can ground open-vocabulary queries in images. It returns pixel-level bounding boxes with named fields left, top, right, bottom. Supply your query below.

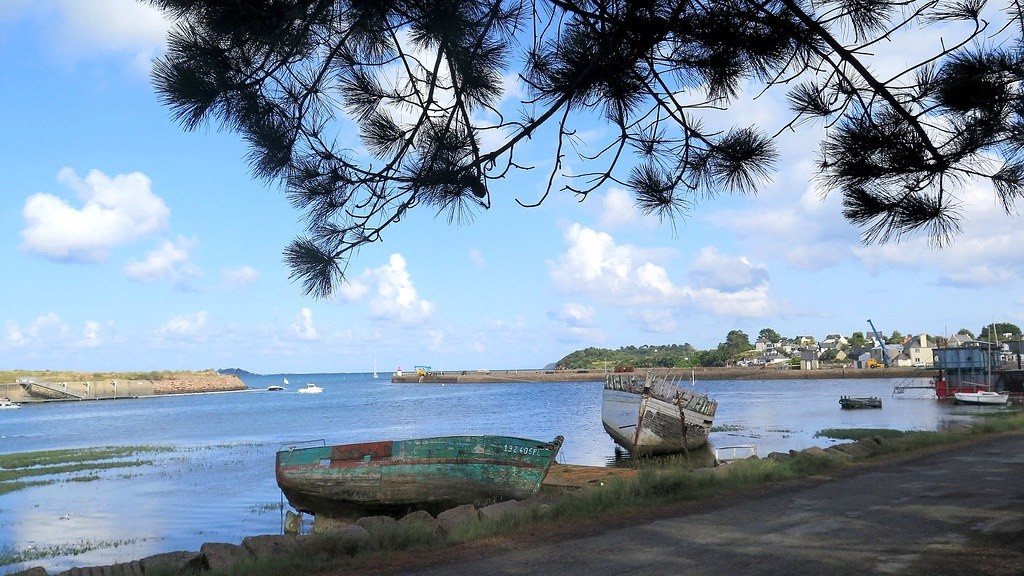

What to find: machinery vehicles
left=866, top=319, right=890, bottom=369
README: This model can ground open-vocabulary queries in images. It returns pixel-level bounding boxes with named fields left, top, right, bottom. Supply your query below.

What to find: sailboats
left=372, top=357, right=378, bottom=379
left=955, top=327, right=1010, bottom=404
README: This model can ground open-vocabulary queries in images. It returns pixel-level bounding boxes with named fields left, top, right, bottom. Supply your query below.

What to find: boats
left=602, top=368, right=718, bottom=455
left=298, top=383, right=324, bottom=393
left=276, top=432, right=564, bottom=515
left=0, top=396, right=21, bottom=409
left=268, top=385, right=283, bottom=392
left=839, top=395, right=882, bottom=408
